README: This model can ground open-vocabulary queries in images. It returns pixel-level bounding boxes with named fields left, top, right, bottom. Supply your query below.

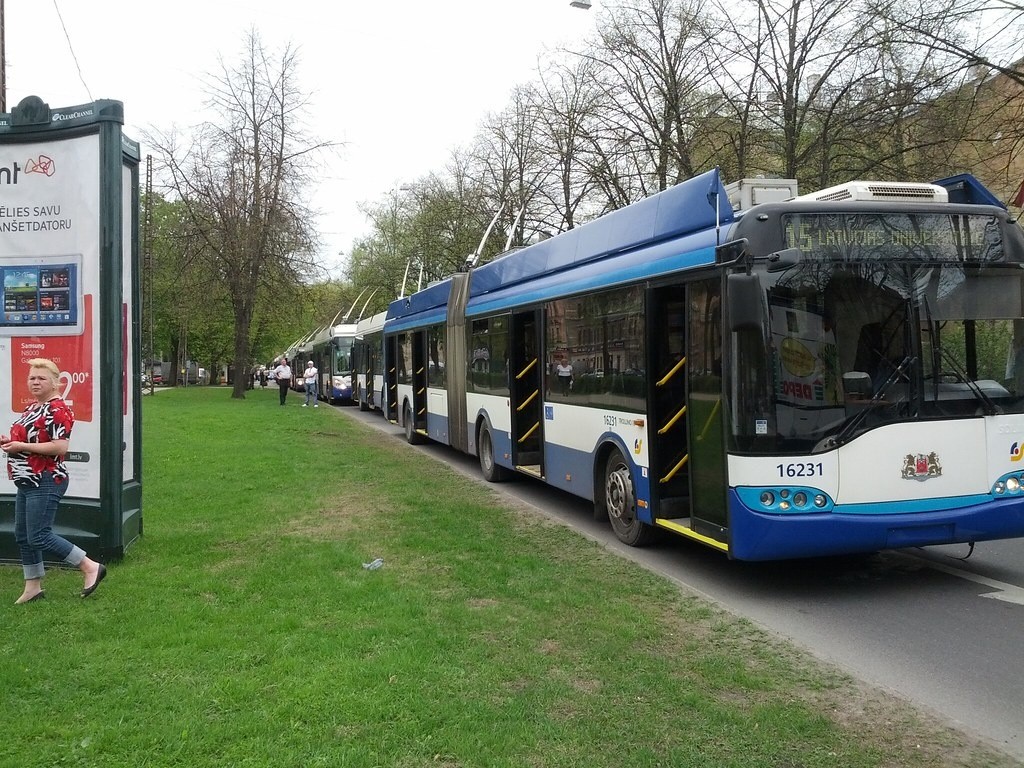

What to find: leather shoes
left=81, top=562, right=106, bottom=600
left=12, top=590, right=45, bottom=605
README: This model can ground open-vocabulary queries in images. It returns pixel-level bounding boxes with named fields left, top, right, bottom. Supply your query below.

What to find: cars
left=151, top=374, right=164, bottom=385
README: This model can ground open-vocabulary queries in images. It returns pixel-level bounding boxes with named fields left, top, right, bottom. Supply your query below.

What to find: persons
left=260, top=368, right=269, bottom=389
left=556, top=359, right=573, bottom=396
left=0, top=358, right=107, bottom=605
left=302, top=361, right=318, bottom=407
left=273, top=359, right=290, bottom=405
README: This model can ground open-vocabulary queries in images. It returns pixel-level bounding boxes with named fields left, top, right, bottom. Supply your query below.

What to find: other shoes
left=563, top=392, right=565, bottom=396
left=301, top=403, right=310, bottom=407
left=280, top=402, right=286, bottom=406
left=313, top=404, right=318, bottom=407
left=566, top=392, right=568, bottom=396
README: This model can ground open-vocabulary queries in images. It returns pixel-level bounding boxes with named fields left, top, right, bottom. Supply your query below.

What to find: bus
left=382, top=165, right=1023, bottom=562
left=266, top=259, right=425, bottom=425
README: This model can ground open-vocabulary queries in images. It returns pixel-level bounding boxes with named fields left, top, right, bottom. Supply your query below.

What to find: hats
left=308, top=360, right=313, bottom=365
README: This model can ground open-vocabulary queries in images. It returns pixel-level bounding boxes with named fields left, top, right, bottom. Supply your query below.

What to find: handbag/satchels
left=262, top=380, right=267, bottom=386
left=569, top=379, right=574, bottom=389
